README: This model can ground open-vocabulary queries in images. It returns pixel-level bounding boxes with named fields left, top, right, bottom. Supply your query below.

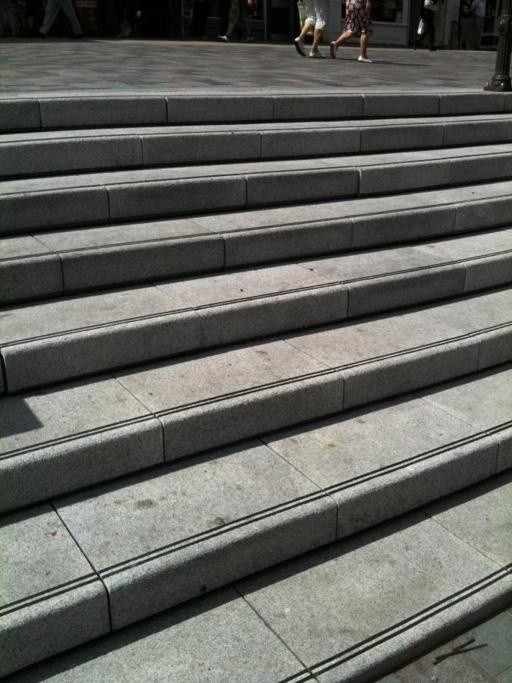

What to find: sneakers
left=357, top=55, right=373, bottom=63
left=294, top=36, right=307, bottom=57
left=330, top=41, right=338, bottom=59
left=308, top=50, right=328, bottom=59
left=217, top=33, right=255, bottom=43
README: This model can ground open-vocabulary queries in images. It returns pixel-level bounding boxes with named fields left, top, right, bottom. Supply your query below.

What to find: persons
left=291, top=0, right=330, bottom=59
left=331, top=0, right=376, bottom=62
left=216, top=0, right=256, bottom=41
left=35, top=0, right=87, bottom=40
left=114, top=0, right=133, bottom=38
left=471, top=0, right=486, bottom=49
left=413, top=0, right=442, bottom=50
left=459, top=0, right=475, bottom=48
